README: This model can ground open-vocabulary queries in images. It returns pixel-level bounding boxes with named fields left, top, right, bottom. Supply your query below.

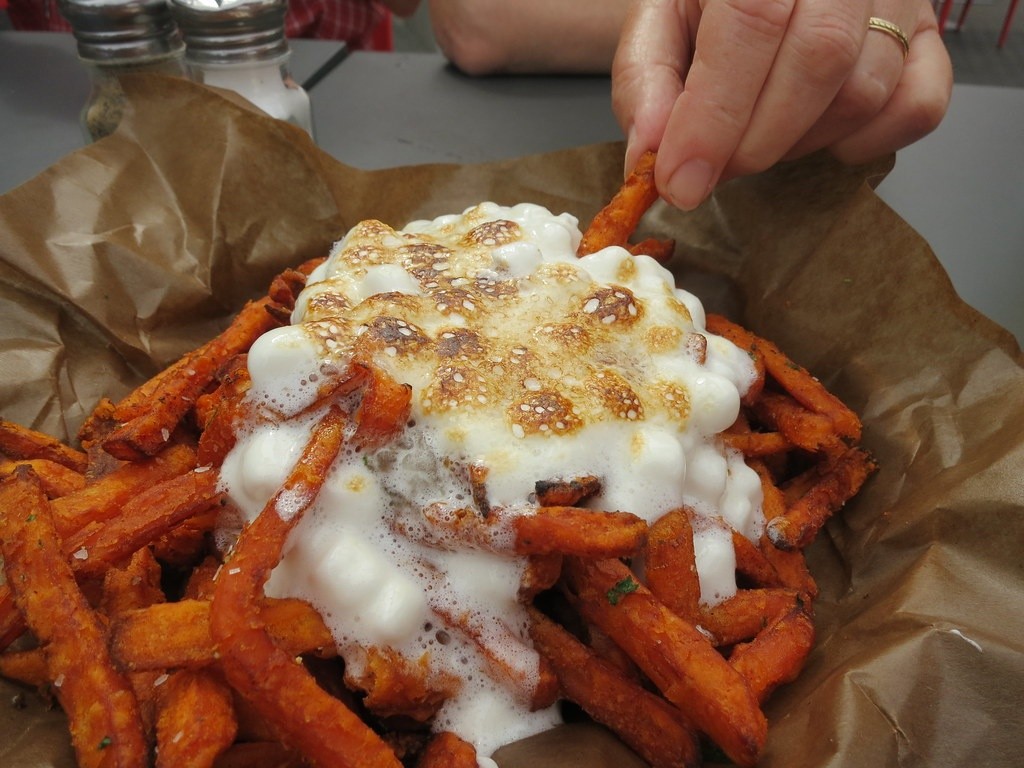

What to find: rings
left=869, top=17, right=910, bottom=61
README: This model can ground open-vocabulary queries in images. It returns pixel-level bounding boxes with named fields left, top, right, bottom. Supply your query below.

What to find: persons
left=426, top=0, right=954, bottom=210
left=0, top=0, right=420, bottom=54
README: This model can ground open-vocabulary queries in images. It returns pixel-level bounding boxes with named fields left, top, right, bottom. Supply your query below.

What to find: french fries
left=0, top=148, right=877, bottom=768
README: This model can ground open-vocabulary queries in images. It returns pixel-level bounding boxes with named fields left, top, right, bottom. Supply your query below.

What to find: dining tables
left=0, top=30, right=1024, bottom=768
